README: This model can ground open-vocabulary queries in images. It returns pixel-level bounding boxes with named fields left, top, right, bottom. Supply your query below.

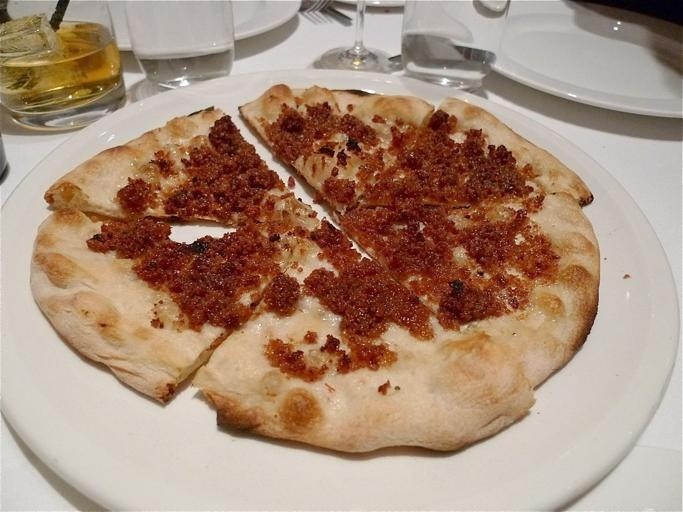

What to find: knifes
left=326, top=4, right=352, bottom=22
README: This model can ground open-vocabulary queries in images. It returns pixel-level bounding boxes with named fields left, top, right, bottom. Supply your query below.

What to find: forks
left=303, top=0, right=346, bottom=17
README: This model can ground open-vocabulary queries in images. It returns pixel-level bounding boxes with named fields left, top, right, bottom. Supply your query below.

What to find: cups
left=0, top=0, right=235, bottom=132
left=401, top=1, right=510, bottom=93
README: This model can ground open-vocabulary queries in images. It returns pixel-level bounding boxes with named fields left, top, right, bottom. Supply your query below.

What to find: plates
left=6, top=1, right=302, bottom=52
left=0, top=68, right=683, bottom=511
left=485, top=1, right=683, bottom=118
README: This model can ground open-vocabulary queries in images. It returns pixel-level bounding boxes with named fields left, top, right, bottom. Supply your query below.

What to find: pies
left=28, top=82, right=601, bottom=452
